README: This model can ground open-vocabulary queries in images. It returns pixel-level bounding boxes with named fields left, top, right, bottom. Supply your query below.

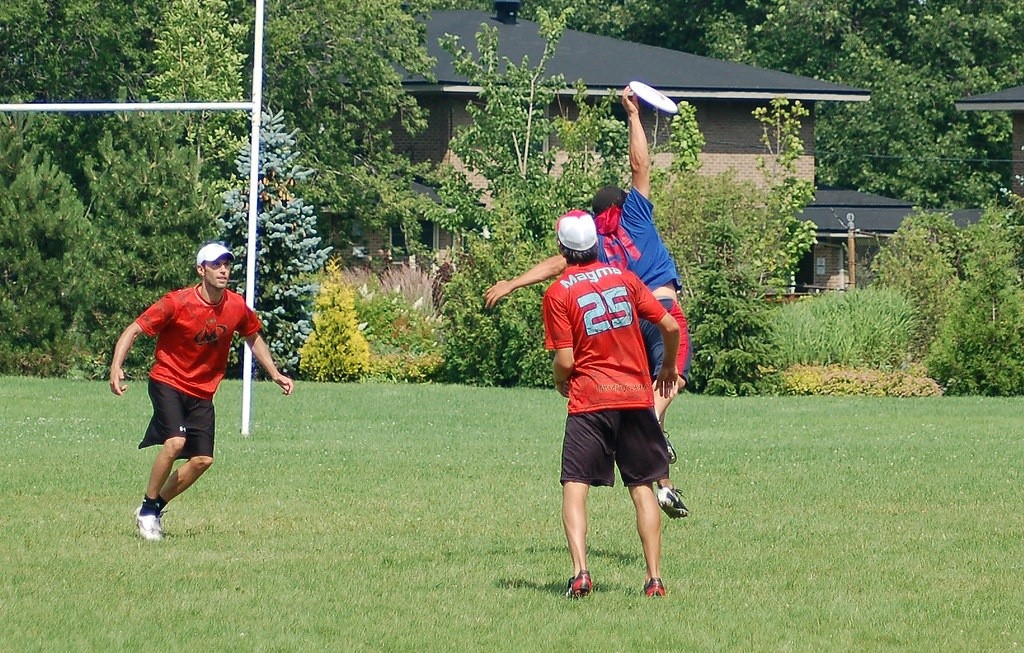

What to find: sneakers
left=662, top=430, right=677, bottom=463
left=643, top=578, right=665, bottom=596
left=134, top=506, right=163, bottom=540
left=657, top=487, right=689, bottom=519
left=565, top=570, right=592, bottom=599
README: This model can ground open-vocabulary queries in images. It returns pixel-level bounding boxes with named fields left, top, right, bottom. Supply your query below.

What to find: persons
left=109, top=243, right=293, bottom=541
left=543, top=211, right=680, bottom=600
left=484, top=86, right=691, bottom=519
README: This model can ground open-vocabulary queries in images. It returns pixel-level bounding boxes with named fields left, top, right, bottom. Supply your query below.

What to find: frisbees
left=629, top=80, right=679, bottom=114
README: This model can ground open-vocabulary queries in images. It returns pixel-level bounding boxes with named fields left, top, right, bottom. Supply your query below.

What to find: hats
left=592, top=185, right=627, bottom=216
left=197, top=244, right=235, bottom=266
left=556, top=209, right=597, bottom=250
